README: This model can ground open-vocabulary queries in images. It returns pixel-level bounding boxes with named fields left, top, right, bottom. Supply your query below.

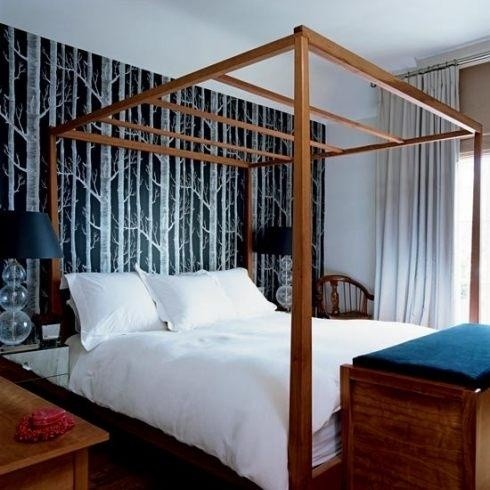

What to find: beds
left=48, top=25, right=483, bottom=490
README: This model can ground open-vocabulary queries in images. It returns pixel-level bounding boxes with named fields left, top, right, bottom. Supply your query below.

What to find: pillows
left=134, top=263, right=277, bottom=331
left=59, top=273, right=169, bottom=351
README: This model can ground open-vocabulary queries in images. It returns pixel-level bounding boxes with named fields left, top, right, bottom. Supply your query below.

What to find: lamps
left=256, top=226, right=294, bottom=311
left=0, top=210, right=64, bottom=354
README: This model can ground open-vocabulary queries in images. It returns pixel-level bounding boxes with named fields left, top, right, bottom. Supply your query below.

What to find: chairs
left=313, top=275, right=374, bottom=320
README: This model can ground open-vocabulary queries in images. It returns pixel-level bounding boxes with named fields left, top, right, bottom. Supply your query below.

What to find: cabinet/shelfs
left=340, top=324, right=490, bottom=490
left=0, top=377, right=109, bottom=490
